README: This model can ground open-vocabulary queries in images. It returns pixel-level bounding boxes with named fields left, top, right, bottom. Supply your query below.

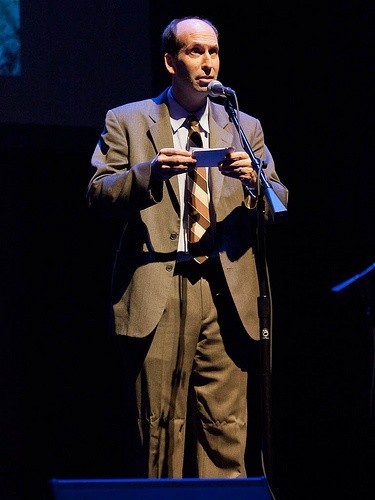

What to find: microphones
left=207, top=80, right=234, bottom=97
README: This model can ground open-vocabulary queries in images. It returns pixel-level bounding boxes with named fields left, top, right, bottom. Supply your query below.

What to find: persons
left=85, top=11, right=291, bottom=480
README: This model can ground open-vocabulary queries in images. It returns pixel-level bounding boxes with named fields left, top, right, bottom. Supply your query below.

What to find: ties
left=187, top=117, right=210, bottom=264
